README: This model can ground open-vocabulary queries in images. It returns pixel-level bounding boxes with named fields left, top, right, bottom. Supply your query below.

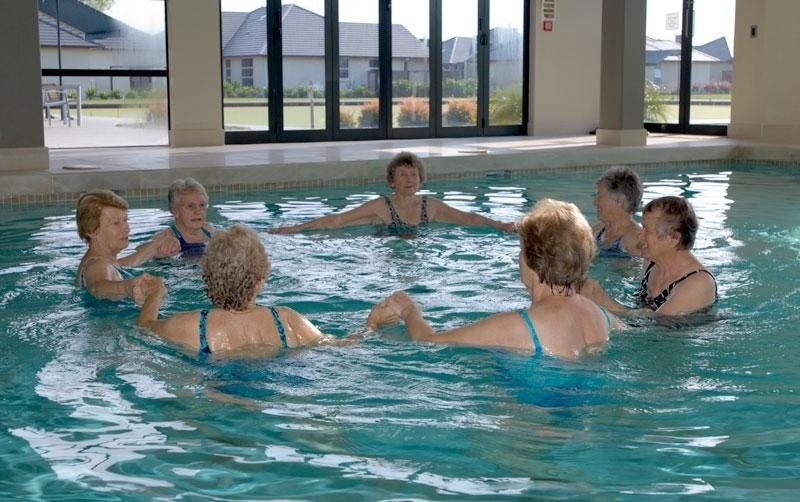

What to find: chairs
left=42, top=84, right=68, bottom=126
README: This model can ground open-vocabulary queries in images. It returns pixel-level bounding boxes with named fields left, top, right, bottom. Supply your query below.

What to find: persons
left=269, top=149, right=519, bottom=243
left=574, top=195, right=719, bottom=325
left=515, top=163, right=641, bottom=258
left=74, top=189, right=183, bottom=304
left=134, top=176, right=286, bottom=260
left=140, top=223, right=403, bottom=360
left=382, top=197, right=627, bottom=358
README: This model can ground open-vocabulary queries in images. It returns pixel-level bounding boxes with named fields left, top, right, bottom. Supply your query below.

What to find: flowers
left=339, top=95, right=476, bottom=129
